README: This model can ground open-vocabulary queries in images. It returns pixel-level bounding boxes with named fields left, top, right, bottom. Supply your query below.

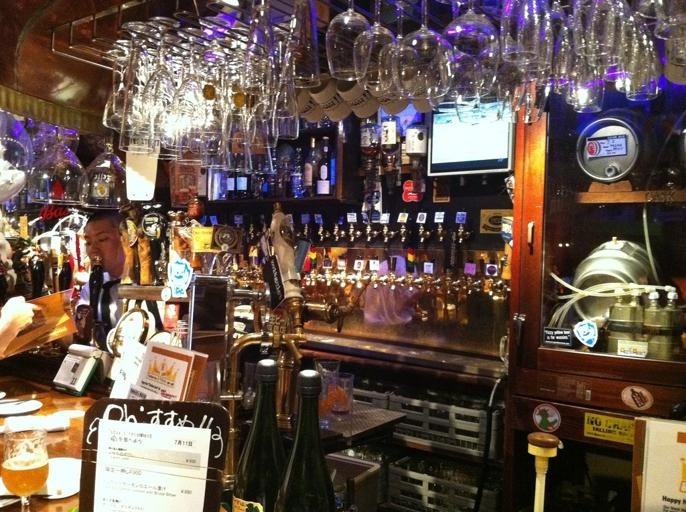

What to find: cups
left=327, top=372, right=355, bottom=426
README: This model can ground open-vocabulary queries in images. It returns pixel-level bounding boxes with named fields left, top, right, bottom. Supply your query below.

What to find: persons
left=0, top=235, right=54, bottom=303
left=374, top=231, right=419, bottom=278
left=71, top=211, right=151, bottom=347
left=183, top=198, right=212, bottom=227
left=0, top=295, right=41, bottom=358
left=105, top=306, right=150, bottom=356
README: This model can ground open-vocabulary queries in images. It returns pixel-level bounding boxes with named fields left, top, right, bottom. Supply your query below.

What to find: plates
left=0, top=398, right=43, bottom=416
left=42, top=455, right=82, bottom=500
left=1, top=475, right=22, bottom=509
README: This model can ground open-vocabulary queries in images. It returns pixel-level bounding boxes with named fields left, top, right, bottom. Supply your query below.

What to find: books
left=0, top=288, right=78, bottom=361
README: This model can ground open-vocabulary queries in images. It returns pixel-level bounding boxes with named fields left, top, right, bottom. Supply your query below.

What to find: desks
left=0, top=370, right=96, bottom=512
left=318, top=395, right=407, bottom=511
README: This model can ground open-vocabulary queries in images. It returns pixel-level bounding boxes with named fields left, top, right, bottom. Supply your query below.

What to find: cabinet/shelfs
left=211, top=119, right=361, bottom=208
left=302, top=312, right=519, bottom=512
left=511, top=80, right=686, bottom=418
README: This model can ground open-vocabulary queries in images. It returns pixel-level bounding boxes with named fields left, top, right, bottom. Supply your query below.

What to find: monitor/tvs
left=427, top=98, right=515, bottom=176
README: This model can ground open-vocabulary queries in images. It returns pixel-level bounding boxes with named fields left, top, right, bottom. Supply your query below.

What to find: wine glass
left=103, top=0, right=305, bottom=175
left=0, top=424, right=50, bottom=512
left=321, top=0, right=686, bottom=128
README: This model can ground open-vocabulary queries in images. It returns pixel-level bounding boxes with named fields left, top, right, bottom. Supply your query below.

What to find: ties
left=101, top=280, right=120, bottom=333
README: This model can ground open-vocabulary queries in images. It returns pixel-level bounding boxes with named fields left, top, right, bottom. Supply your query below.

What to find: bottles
left=270, top=366, right=336, bottom=512
left=291, top=249, right=510, bottom=338
left=225, top=129, right=334, bottom=199
left=234, top=357, right=290, bottom=512
